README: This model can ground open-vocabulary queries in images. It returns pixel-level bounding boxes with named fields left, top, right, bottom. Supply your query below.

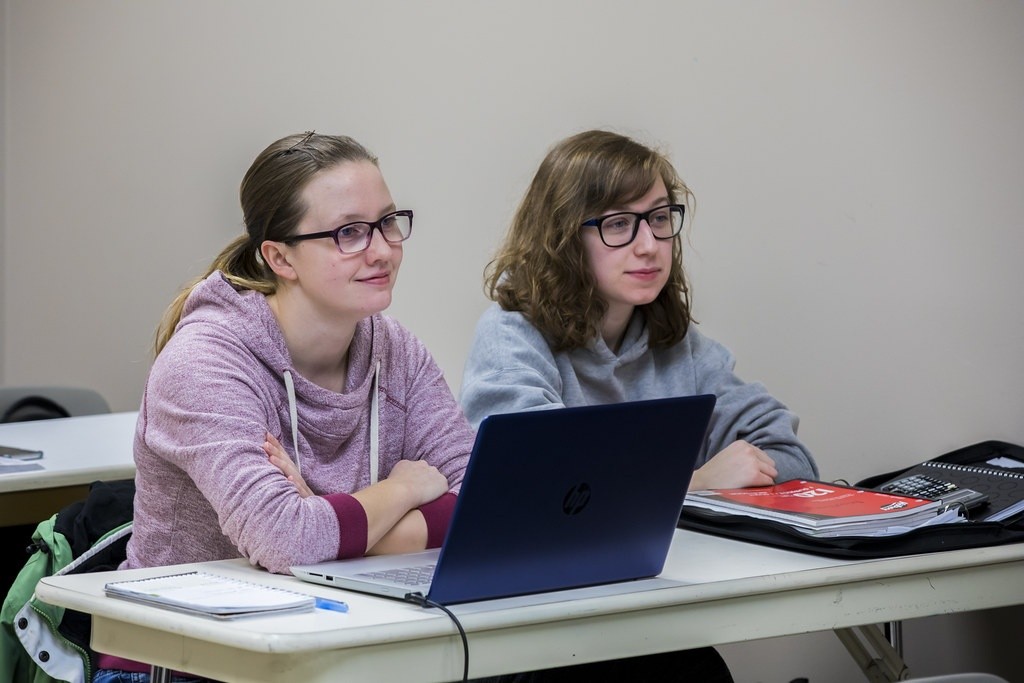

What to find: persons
left=91, top=132, right=477, bottom=682
left=459, top=130, right=820, bottom=682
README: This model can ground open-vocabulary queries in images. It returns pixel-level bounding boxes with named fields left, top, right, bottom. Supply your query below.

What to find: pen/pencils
left=314, top=596, right=350, bottom=612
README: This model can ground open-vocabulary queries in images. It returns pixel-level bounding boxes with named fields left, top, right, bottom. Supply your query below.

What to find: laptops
left=289, top=393, right=717, bottom=610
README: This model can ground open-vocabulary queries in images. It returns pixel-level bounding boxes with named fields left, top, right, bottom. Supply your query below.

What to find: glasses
left=581, top=203, right=686, bottom=247
left=275, top=210, right=414, bottom=256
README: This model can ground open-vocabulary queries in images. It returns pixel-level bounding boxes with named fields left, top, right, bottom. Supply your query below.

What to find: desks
left=0, top=410, right=137, bottom=527
left=35, top=528, right=1024, bottom=683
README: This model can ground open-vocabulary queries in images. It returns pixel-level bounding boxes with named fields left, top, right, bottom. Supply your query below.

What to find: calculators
left=874, top=472, right=989, bottom=511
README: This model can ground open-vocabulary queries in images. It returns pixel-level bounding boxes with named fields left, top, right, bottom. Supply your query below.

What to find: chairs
left=0, top=388, right=103, bottom=424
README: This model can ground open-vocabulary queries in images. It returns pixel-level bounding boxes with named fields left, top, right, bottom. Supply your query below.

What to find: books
left=103, top=571, right=316, bottom=622
left=684, top=476, right=945, bottom=540
